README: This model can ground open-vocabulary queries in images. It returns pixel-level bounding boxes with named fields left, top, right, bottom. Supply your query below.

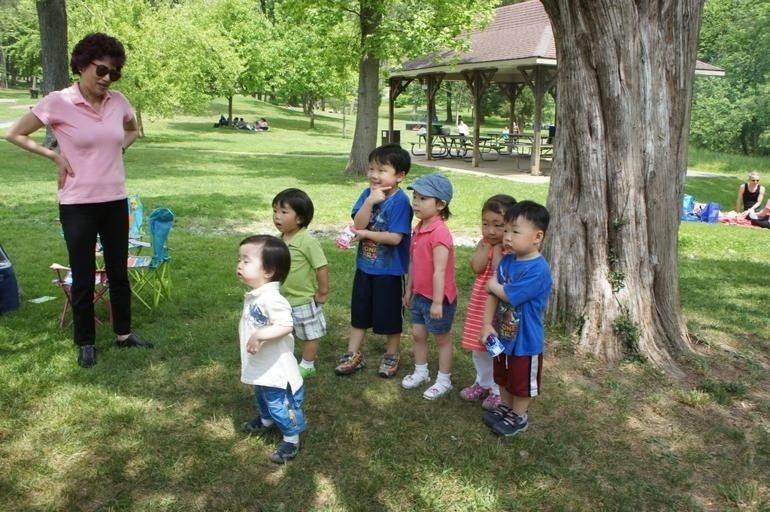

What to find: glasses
left=89, top=59, right=121, bottom=81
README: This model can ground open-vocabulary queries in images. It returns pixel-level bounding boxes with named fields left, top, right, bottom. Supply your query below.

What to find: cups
left=337, top=225, right=354, bottom=249
left=484, top=333, right=506, bottom=358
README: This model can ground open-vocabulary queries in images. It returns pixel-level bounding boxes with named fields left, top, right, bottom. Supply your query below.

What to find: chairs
left=93, top=200, right=145, bottom=255
left=50, top=263, right=113, bottom=330
left=126, top=208, right=174, bottom=308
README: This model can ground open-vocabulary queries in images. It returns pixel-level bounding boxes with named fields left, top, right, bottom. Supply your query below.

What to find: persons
left=272, top=187, right=329, bottom=379
left=752, top=199, right=770, bottom=228
left=500, top=126, right=510, bottom=142
left=334, top=143, right=412, bottom=377
left=480, top=199, right=552, bottom=437
left=236, top=235, right=306, bottom=464
left=460, top=194, right=517, bottom=410
left=735, top=172, right=766, bottom=220
left=512, top=122, right=520, bottom=144
left=458, top=119, right=469, bottom=147
left=401, top=174, right=458, bottom=401
left=6, top=33, right=153, bottom=367
left=219, top=115, right=268, bottom=132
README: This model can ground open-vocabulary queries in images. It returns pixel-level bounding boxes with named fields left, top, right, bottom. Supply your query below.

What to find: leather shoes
left=78, top=346, right=98, bottom=368
left=116, top=334, right=153, bottom=349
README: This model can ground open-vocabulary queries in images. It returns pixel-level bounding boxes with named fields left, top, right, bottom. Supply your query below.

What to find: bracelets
left=122, top=147, right=126, bottom=156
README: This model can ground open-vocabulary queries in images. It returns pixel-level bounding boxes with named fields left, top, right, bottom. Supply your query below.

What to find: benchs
left=408, top=130, right=553, bottom=169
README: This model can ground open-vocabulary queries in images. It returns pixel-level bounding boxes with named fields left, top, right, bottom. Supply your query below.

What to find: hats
left=405, top=174, right=452, bottom=204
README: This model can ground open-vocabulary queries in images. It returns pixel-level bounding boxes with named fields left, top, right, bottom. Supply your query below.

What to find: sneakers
left=299, top=362, right=317, bottom=379
left=481, top=388, right=502, bottom=411
left=458, top=380, right=487, bottom=402
left=423, top=379, right=453, bottom=400
left=481, top=405, right=510, bottom=427
left=377, top=353, right=401, bottom=377
left=401, top=372, right=430, bottom=388
left=334, top=352, right=369, bottom=375
left=271, top=439, right=301, bottom=464
left=240, top=416, right=277, bottom=432
left=492, top=412, right=528, bottom=437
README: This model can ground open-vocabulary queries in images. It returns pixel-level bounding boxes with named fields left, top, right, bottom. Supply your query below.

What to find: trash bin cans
left=549, top=126, right=556, bottom=142
left=382, top=130, right=400, bottom=146
left=432, top=124, right=442, bottom=135
